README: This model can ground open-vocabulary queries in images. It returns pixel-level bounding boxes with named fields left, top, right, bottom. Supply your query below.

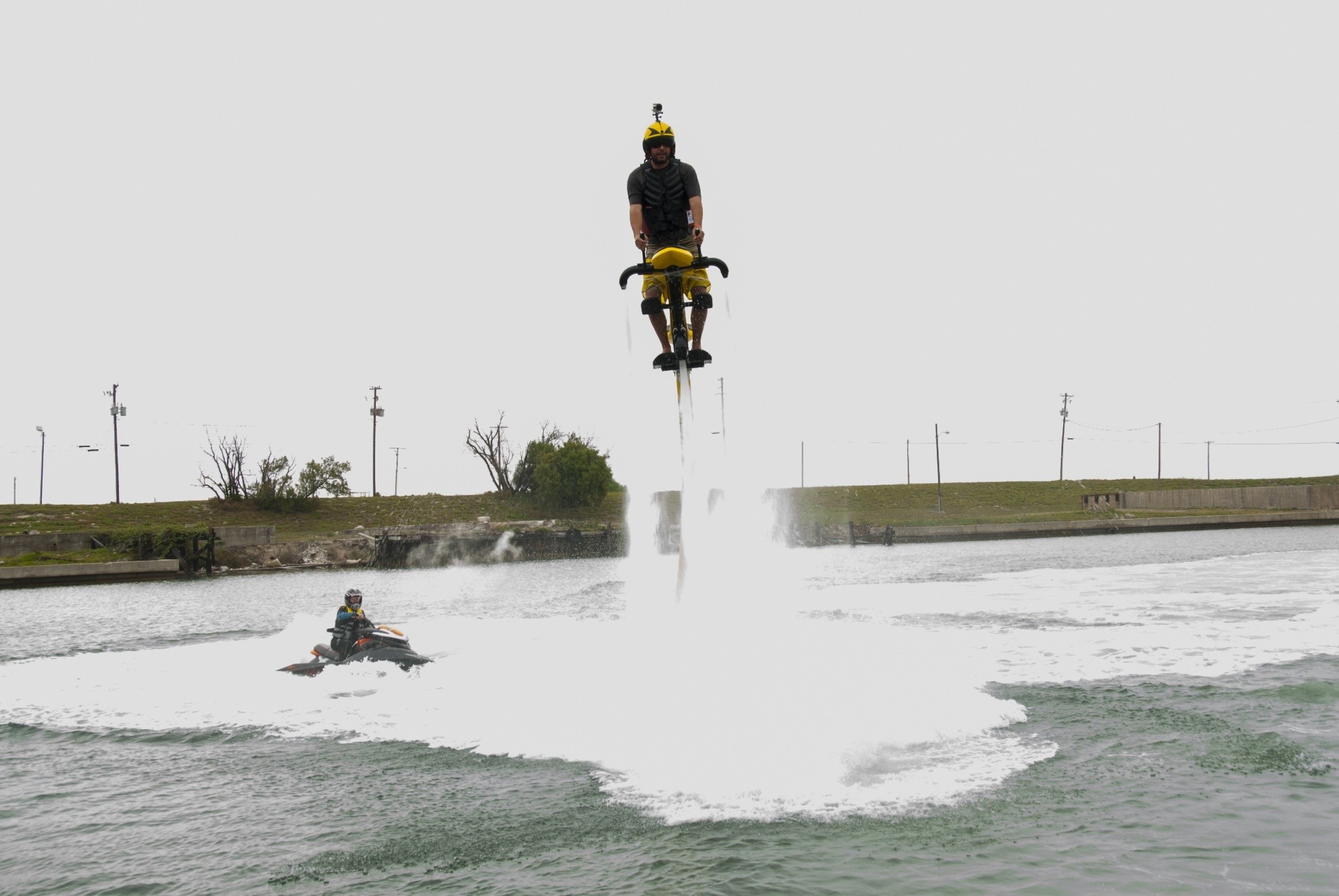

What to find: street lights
left=36, top=425, right=45, bottom=505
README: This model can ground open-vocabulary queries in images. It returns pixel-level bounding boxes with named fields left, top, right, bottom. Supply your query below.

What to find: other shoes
left=653, top=353, right=675, bottom=369
left=688, top=349, right=713, bottom=364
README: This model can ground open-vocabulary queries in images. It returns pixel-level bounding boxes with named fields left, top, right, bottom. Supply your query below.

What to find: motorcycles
left=275, top=613, right=435, bottom=678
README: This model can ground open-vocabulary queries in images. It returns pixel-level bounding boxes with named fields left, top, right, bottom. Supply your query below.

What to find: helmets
left=642, top=121, right=675, bottom=160
left=344, top=589, right=362, bottom=611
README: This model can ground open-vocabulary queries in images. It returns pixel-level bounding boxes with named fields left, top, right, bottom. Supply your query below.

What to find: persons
left=331, top=588, right=365, bottom=653
left=627, top=121, right=712, bottom=368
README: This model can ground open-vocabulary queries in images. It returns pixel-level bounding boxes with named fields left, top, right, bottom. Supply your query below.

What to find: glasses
left=646, top=136, right=675, bottom=148
